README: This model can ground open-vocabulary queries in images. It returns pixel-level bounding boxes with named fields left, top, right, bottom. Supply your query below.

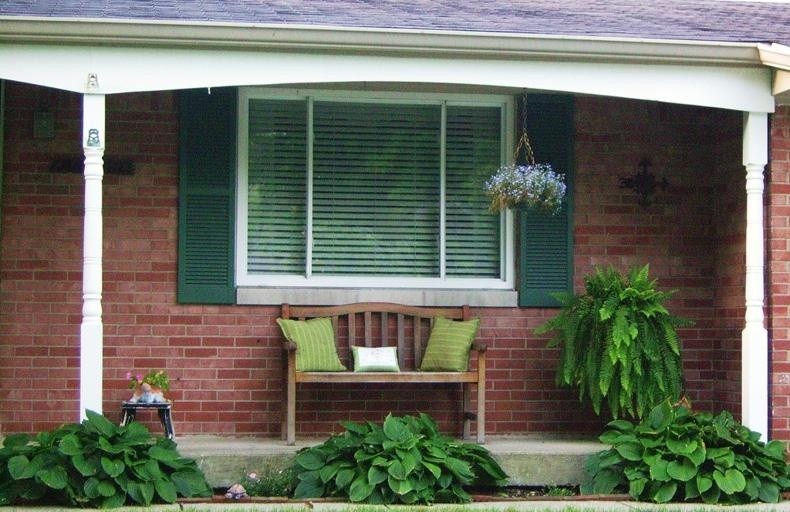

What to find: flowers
left=484, top=158, right=566, bottom=215
left=125, top=370, right=170, bottom=390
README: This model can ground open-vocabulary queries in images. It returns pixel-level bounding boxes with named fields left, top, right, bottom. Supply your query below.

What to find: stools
left=117, top=399, right=176, bottom=442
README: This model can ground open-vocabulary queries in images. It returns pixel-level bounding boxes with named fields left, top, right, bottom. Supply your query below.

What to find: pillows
left=351, top=346, right=402, bottom=374
left=419, top=314, right=481, bottom=373
left=275, top=316, right=349, bottom=373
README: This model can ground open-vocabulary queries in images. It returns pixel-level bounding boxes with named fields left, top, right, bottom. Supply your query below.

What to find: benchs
left=279, top=301, right=488, bottom=445
